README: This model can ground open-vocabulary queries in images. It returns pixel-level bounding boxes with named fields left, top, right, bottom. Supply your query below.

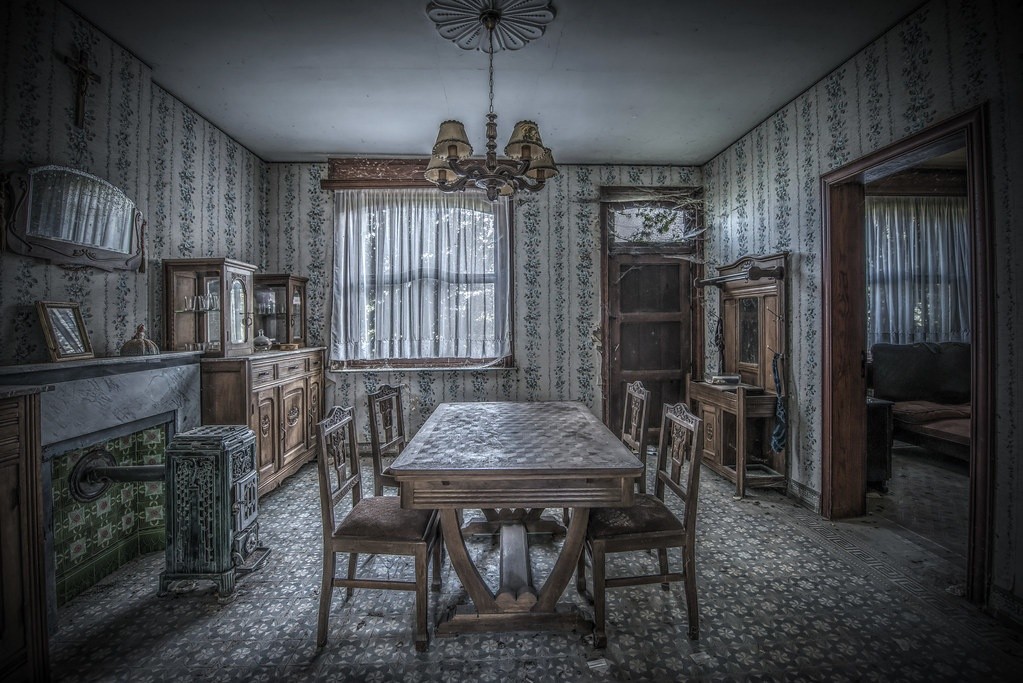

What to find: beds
left=871, top=340, right=972, bottom=465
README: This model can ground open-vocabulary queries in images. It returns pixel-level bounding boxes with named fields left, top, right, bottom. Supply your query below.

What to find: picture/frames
left=34, top=299, right=96, bottom=363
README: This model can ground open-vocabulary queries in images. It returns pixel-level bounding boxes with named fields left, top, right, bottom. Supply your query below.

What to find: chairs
left=558, top=380, right=650, bottom=568
left=368, top=385, right=446, bottom=591
left=314, top=406, right=449, bottom=655
left=581, top=403, right=705, bottom=647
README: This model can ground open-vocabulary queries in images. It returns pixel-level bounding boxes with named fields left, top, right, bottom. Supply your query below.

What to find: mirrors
left=736, top=296, right=761, bottom=371
left=0, top=165, right=147, bottom=275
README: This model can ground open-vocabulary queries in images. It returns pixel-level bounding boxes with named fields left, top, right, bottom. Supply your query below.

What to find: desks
left=381, top=402, right=645, bottom=638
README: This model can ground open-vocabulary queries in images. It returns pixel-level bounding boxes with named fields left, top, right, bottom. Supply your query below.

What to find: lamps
left=423, top=1, right=560, bottom=204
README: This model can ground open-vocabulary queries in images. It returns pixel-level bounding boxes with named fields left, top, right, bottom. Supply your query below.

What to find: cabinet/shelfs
left=868, top=397, right=895, bottom=491
left=0, top=383, right=54, bottom=683
left=684, top=250, right=790, bottom=497
left=164, top=258, right=327, bottom=498
